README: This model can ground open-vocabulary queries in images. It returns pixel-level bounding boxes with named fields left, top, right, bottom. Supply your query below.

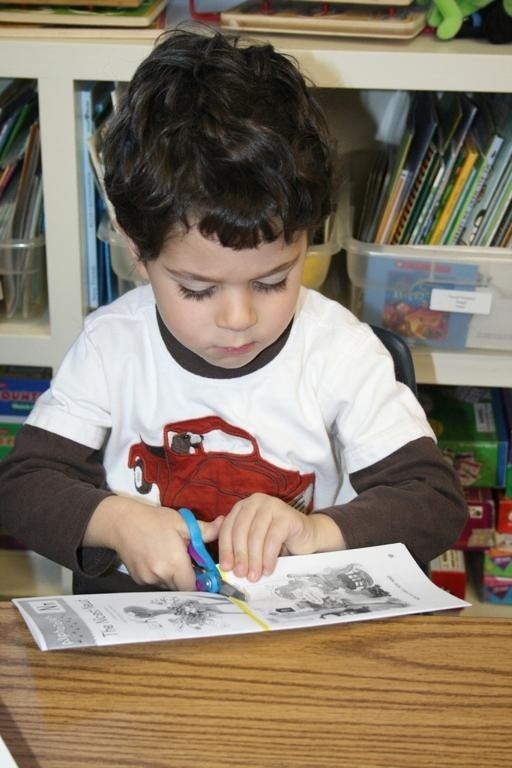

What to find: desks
left=1, top=600, right=512, bottom=766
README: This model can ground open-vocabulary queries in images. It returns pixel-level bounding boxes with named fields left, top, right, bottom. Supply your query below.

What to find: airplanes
left=392, top=301, right=443, bottom=340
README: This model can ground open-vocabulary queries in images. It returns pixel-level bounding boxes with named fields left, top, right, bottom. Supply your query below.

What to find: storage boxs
left=0, top=233, right=48, bottom=325
left=333, top=138, right=511, bottom=352
left=94, top=175, right=349, bottom=292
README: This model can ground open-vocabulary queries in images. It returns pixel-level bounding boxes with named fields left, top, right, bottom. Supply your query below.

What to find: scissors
left=177, top=508, right=246, bottom=602
